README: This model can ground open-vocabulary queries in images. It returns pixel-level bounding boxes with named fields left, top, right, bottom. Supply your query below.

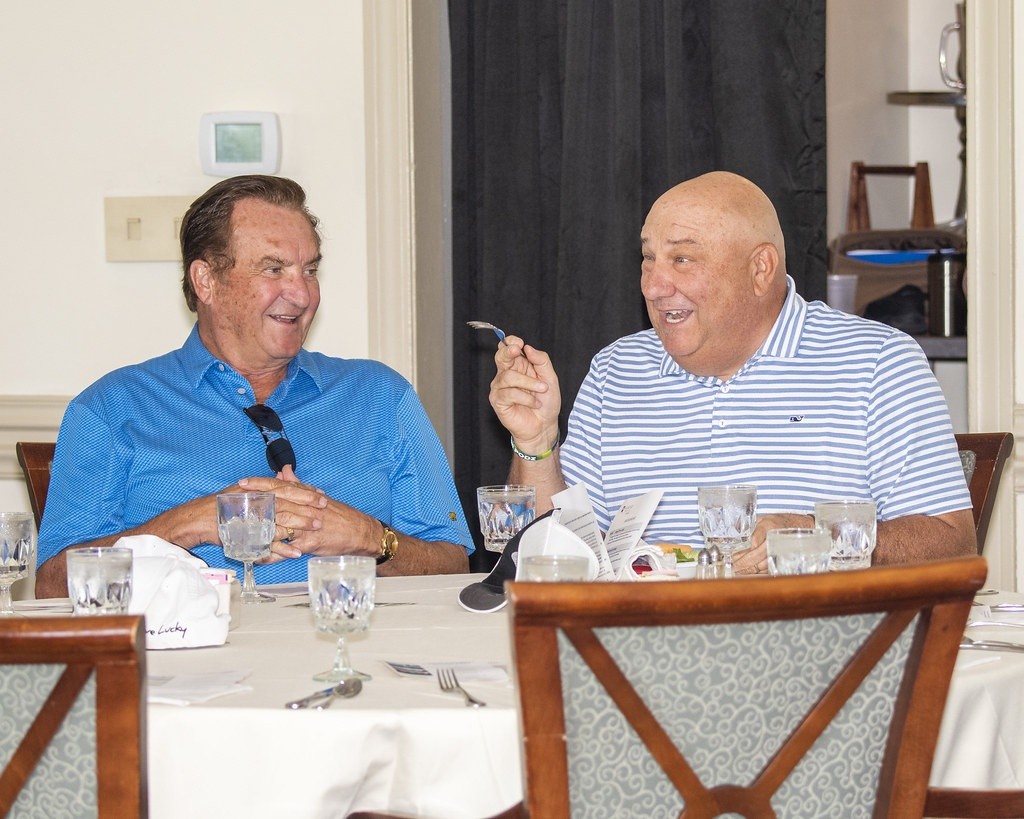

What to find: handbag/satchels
left=863, top=284, right=928, bottom=337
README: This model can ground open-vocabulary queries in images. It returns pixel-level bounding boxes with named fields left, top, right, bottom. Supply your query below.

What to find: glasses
left=243, top=404, right=296, bottom=472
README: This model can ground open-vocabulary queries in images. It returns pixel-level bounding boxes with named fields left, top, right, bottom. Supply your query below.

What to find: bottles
left=696, top=547, right=735, bottom=578
left=201, top=569, right=241, bottom=631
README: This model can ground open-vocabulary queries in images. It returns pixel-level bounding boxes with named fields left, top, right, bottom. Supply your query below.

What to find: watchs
left=376, top=520, right=399, bottom=565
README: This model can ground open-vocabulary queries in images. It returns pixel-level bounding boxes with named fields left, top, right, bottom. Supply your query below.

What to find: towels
left=109, top=534, right=233, bottom=651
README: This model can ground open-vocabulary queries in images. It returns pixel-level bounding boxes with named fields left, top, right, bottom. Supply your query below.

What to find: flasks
left=928, top=254, right=965, bottom=337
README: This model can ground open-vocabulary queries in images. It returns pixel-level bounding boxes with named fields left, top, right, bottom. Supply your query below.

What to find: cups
left=524, top=556, right=589, bottom=584
left=827, top=274, right=858, bottom=314
left=677, top=561, right=697, bottom=579
left=767, top=527, right=831, bottom=576
left=477, top=486, right=536, bottom=552
left=815, top=500, right=876, bottom=571
left=67, top=547, right=133, bottom=615
left=698, top=484, right=757, bottom=559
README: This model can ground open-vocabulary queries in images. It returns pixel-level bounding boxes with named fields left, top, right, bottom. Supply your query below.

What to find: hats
left=457, top=508, right=561, bottom=614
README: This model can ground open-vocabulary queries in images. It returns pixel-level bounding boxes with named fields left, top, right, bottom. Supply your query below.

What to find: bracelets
left=511, top=429, right=561, bottom=461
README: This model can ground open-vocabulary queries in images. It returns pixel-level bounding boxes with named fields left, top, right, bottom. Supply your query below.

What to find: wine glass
left=217, top=493, right=276, bottom=604
left=0, top=513, right=31, bottom=619
left=307, top=555, right=376, bottom=680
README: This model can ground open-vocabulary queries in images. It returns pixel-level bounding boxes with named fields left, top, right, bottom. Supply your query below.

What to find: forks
left=437, top=668, right=486, bottom=708
left=467, top=320, right=526, bottom=359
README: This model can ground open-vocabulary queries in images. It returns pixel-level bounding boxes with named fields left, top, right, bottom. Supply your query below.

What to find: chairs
left=0, top=436, right=1016, bottom=819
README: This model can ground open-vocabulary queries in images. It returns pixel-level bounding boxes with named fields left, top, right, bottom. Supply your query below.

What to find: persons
left=489, top=171, right=977, bottom=576
left=34, top=174, right=477, bottom=599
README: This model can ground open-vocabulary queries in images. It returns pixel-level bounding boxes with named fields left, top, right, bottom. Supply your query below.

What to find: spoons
left=284, top=679, right=362, bottom=708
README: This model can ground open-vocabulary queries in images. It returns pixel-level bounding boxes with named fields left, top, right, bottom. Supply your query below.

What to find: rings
left=287, top=527, right=294, bottom=540
left=754, top=565, right=760, bottom=574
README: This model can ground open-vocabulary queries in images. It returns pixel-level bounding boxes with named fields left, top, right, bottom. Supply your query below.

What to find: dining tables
left=0, top=573, right=1024, bottom=819
